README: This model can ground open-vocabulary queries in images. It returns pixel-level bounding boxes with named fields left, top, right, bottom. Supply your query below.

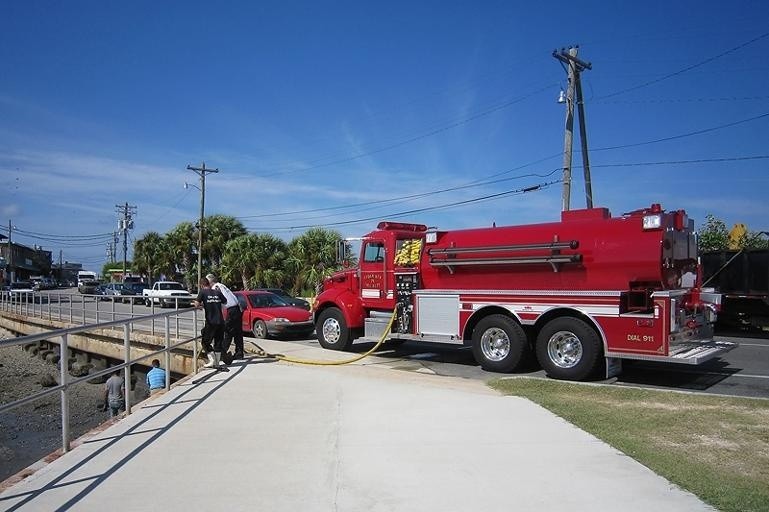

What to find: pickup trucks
left=142, top=281, right=194, bottom=308
left=78, top=281, right=99, bottom=295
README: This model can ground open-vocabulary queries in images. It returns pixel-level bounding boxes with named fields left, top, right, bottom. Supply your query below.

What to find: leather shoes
left=233, top=354, right=244, bottom=360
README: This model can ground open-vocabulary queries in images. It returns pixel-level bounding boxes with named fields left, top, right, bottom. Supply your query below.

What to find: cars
left=221, top=290, right=315, bottom=340
left=104, top=283, right=136, bottom=303
left=1, top=282, right=36, bottom=300
left=26, top=275, right=75, bottom=290
left=254, top=288, right=311, bottom=312
left=92, top=284, right=109, bottom=301
left=121, top=281, right=149, bottom=305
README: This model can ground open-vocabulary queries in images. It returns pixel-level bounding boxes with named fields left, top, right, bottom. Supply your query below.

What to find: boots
left=215, top=351, right=221, bottom=365
left=203, top=351, right=217, bottom=368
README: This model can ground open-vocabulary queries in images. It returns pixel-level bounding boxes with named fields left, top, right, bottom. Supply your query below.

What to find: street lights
left=183, top=180, right=202, bottom=193
left=8, top=218, right=20, bottom=263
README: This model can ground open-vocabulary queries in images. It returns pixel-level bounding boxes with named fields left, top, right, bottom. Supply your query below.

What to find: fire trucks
left=312, top=206, right=739, bottom=382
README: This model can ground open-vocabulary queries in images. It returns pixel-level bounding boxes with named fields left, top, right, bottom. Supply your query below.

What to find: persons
left=190, top=277, right=228, bottom=368
left=103, top=369, right=125, bottom=418
left=206, top=274, right=244, bottom=359
left=146, top=359, right=166, bottom=397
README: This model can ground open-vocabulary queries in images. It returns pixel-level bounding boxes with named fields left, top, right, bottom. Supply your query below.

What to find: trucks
left=77, top=270, right=97, bottom=292
